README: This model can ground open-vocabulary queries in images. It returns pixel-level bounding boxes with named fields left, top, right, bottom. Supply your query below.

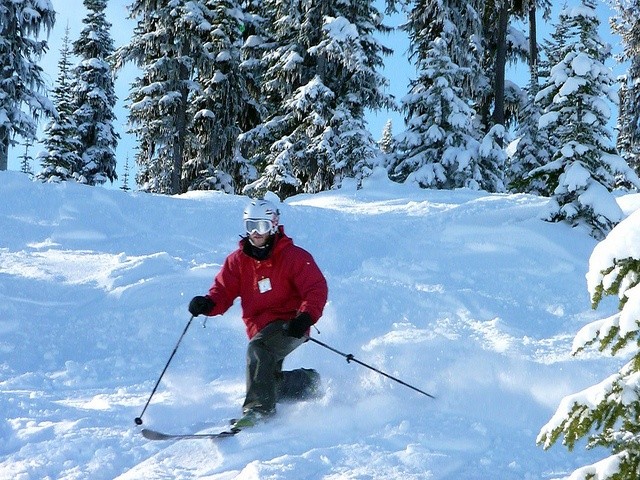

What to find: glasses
left=244, top=220, right=272, bottom=235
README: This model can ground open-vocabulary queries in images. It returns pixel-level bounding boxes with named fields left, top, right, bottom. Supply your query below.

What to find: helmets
left=244, top=200, right=279, bottom=233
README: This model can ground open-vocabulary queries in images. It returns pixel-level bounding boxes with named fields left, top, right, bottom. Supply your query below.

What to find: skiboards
left=141, top=426, right=239, bottom=441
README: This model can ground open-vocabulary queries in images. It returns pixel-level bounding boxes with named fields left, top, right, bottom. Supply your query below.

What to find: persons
left=188, top=197, right=328, bottom=433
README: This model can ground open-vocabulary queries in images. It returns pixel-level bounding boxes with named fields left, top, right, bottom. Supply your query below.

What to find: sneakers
left=236, top=403, right=278, bottom=430
left=299, top=368, right=321, bottom=401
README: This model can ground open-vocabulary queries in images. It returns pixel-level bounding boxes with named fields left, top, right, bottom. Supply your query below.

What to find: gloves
left=189, top=296, right=213, bottom=317
left=282, top=313, right=311, bottom=339
